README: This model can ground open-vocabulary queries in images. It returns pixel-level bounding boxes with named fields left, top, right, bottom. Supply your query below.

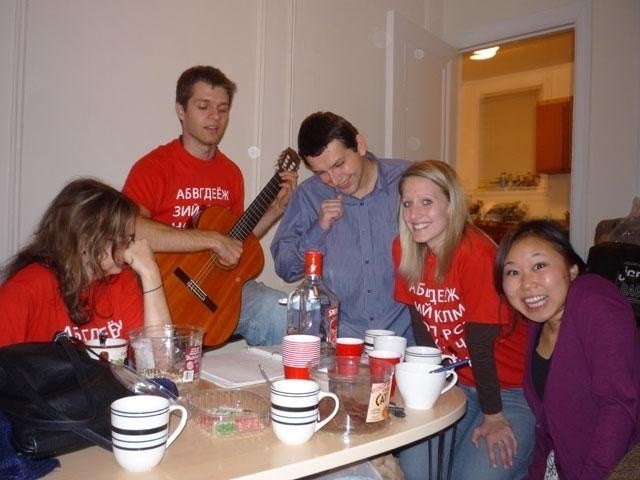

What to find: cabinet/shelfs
left=471, top=215, right=569, bottom=244
left=464, top=173, right=550, bottom=195
left=535, top=96, right=574, bottom=173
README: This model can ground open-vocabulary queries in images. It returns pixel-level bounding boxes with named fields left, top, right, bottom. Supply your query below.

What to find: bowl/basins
left=85, top=339, right=128, bottom=364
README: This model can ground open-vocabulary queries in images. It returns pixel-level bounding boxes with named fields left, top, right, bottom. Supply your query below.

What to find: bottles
left=287, top=250, right=340, bottom=359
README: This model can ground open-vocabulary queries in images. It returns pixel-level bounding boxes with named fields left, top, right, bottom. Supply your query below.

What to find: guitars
left=149, top=146, right=301, bottom=345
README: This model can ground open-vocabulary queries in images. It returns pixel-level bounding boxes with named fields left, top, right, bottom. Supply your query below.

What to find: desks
left=22, top=334, right=468, bottom=480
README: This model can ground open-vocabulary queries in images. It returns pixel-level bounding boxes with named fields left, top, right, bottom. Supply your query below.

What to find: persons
left=120, top=66, right=299, bottom=346
left=271, top=112, right=420, bottom=350
left=390, top=159, right=536, bottom=479
left=0, top=176, right=175, bottom=373
left=494, top=219, right=640, bottom=479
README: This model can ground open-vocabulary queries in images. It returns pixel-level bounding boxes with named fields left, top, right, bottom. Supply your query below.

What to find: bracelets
left=143, top=285, right=163, bottom=294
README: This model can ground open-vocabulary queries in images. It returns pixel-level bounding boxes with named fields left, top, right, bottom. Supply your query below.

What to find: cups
left=336, top=335, right=362, bottom=375
left=376, top=337, right=406, bottom=363
left=270, top=379, right=339, bottom=448
left=364, top=329, right=392, bottom=356
left=283, top=335, right=319, bottom=379
left=111, top=396, right=188, bottom=471
left=394, top=363, right=458, bottom=411
left=405, top=347, right=454, bottom=378
left=369, top=351, right=400, bottom=396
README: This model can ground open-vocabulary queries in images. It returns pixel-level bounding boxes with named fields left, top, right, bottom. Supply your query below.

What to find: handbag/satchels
left=1, top=330, right=141, bottom=461
